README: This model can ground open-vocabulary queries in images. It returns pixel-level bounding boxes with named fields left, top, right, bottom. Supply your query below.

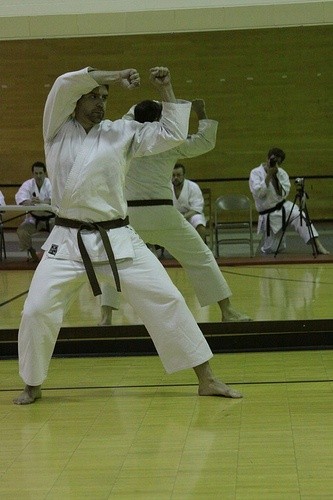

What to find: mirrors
left=0, top=23, right=332, bottom=337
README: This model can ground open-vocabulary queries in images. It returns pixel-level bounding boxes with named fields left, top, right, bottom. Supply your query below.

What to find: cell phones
left=270, top=158, right=276, bottom=167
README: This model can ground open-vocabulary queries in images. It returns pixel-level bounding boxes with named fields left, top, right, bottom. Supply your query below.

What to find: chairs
left=212, top=192, right=255, bottom=258
left=199, top=188, right=213, bottom=253
left=25, top=210, right=54, bottom=262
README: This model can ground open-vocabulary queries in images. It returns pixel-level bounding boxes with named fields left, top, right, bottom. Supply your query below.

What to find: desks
left=0, top=202, right=56, bottom=259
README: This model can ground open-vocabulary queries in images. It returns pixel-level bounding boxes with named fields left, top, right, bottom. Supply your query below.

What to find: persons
left=248, top=148, right=331, bottom=256
left=95, top=66, right=255, bottom=325
left=170, top=163, right=206, bottom=245
left=0, top=191, right=6, bottom=227
left=15, top=162, right=59, bottom=262
left=14, top=64, right=242, bottom=402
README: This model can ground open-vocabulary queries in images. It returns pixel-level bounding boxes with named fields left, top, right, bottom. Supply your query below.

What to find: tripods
left=274, top=190, right=320, bottom=258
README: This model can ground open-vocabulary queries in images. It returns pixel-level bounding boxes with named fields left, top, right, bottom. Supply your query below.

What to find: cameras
left=295, top=178, right=304, bottom=191
